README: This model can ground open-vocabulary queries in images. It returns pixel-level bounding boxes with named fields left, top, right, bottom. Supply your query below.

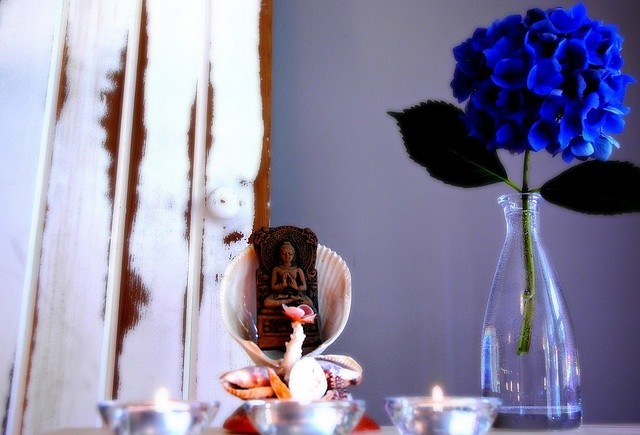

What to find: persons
left=261, top=242, right=313, bottom=315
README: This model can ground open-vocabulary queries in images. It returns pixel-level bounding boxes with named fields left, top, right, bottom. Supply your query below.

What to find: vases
left=480, top=190, right=584, bottom=429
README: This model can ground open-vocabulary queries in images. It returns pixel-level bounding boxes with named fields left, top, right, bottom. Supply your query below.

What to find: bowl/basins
left=246, top=399, right=368, bottom=434
left=97, top=400, right=221, bottom=434
left=387, top=396, right=503, bottom=434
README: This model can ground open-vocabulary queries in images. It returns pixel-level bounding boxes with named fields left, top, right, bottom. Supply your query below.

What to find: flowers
left=383, top=1, right=640, bottom=353
